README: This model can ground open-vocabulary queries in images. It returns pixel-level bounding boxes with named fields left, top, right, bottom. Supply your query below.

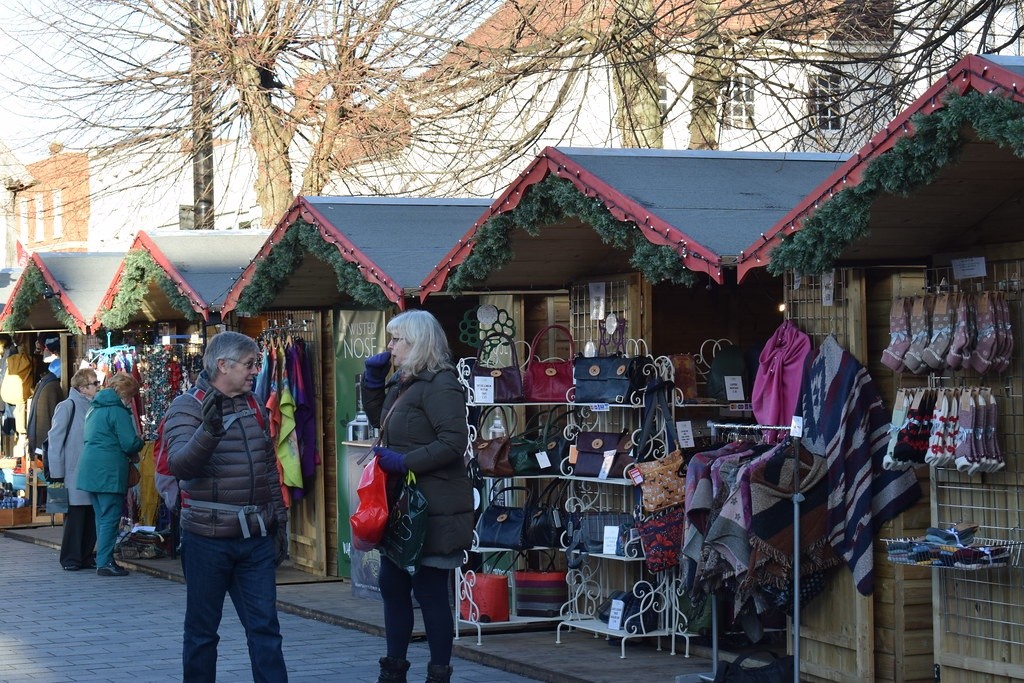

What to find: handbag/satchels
left=374, top=470, right=430, bottom=577
left=42, top=399, right=76, bottom=482
left=459, top=325, right=749, bottom=637
left=46, top=481, right=69, bottom=513
left=350, top=455, right=390, bottom=552
left=128, top=462, right=141, bottom=487
left=2, top=354, right=38, bottom=404
left=713, top=648, right=794, bottom=683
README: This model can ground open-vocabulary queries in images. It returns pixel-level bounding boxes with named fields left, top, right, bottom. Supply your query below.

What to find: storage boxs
left=0, top=469, right=26, bottom=490
left=879, top=536, right=1024, bottom=572
left=0, top=506, right=31, bottom=527
left=53, top=513, right=65, bottom=526
left=120, top=544, right=156, bottom=560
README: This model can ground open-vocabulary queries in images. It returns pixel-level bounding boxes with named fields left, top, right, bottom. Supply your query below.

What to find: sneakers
left=97, top=561, right=129, bottom=576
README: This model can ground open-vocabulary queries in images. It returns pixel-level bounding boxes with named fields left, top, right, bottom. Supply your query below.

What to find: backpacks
left=154, top=387, right=269, bottom=515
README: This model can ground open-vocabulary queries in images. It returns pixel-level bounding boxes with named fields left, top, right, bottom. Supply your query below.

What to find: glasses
left=392, top=337, right=406, bottom=344
left=228, top=357, right=260, bottom=369
left=80, top=381, right=100, bottom=386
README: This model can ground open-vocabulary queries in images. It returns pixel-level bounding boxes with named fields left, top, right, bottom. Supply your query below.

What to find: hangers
left=721, top=423, right=792, bottom=448
left=88, top=331, right=136, bottom=364
left=262, top=325, right=293, bottom=350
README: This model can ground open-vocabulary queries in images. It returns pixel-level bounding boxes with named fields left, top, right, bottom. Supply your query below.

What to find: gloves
left=373, top=446, right=407, bottom=474
left=271, top=522, right=287, bottom=566
left=364, top=351, right=391, bottom=388
left=201, top=387, right=226, bottom=436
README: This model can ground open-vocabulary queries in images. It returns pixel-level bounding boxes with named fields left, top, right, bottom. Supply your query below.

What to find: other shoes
left=90, top=563, right=97, bottom=568
left=64, top=564, right=79, bottom=570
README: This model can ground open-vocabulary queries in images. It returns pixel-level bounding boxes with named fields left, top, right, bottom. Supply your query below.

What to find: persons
left=360, top=311, right=475, bottom=683
left=162, top=332, right=289, bottom=683
left=75, top=372, right=145, bottom=577
left=48, top=368, right=97, bottom=571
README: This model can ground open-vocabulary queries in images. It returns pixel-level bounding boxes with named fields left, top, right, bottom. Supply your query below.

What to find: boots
left=424, top=662, right=453, bottom=683
left=375, top=656, right=411, bottom=683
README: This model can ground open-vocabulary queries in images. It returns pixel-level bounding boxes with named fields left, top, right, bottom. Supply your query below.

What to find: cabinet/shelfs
left=341, top=438, right=421, bottom=609
left=556, top=339, right=686, bottom=659
left=28, top=454, right=54, bottom=523
left=455, top=340, right=568, bottom=645
left=634, top=339, right=732, bottom=443
